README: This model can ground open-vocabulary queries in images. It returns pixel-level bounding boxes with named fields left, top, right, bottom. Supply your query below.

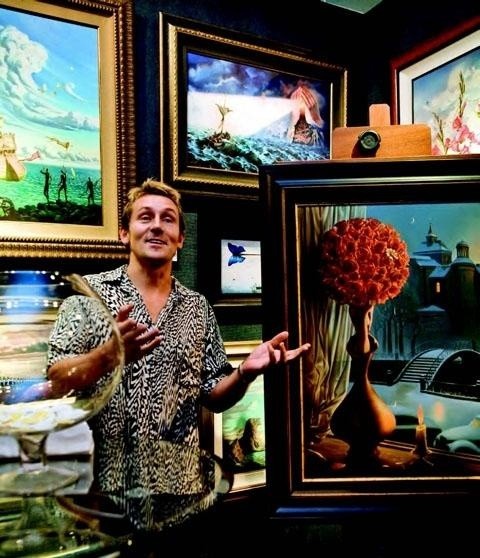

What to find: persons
left=45, top=177, right=311, bottom=558
left=56, top=170, right=67, bottom=202
left=40, top=168, right=52, bottom=206
left=279, top=74, right=323, bottom=146
left=85, top=177, right=94, bottom=206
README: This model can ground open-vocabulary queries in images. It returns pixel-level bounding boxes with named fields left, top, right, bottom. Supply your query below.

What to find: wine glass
left=0, top=268, right=125, bottom=500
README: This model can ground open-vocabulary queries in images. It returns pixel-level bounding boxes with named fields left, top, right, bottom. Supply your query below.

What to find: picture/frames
left=391, top=21, right=476, bottom=154
left=0, top=0, right=134, bottom=253
left=193, top=211, right=259, bottom=323
left=256, top=153, right=479, bottom=522
left=206, top=341, right=269, bottom=500
left=0, top=258, right=141, bottom=515
left=156, top=11, right=354, bottom=188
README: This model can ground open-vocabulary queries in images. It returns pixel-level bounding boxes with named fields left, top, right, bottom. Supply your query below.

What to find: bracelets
left=238, top=365, right=256, bottom=383
